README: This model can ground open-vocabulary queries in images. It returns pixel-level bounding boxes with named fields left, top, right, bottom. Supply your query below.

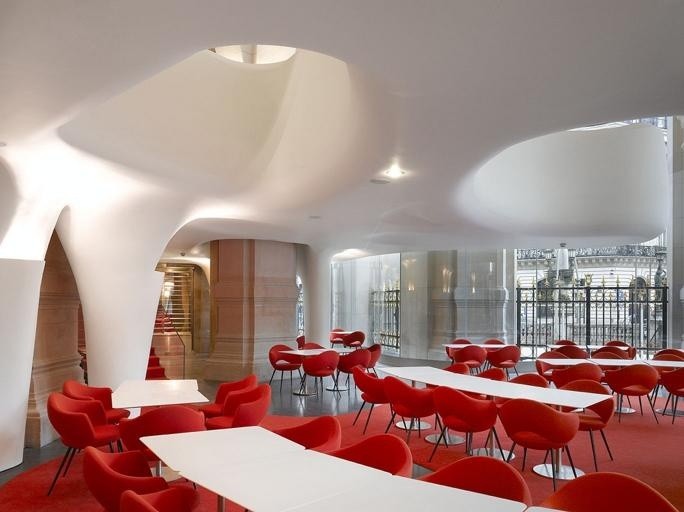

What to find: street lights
left=654, top=243, right=665, bottom=317
left=540, top=248, right=581, bottom=343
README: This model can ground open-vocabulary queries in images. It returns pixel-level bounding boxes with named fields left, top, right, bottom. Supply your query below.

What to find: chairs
left=274, top=415, right=342, bottom=453
left=443, top=339, right=521, bottom=381
left=265, top=329, right=381, bottom=400
left=330, top=434, right=413, bottom=477
left=535, top=340, right=684, bottom=425
left=45, top=374, right=271, bottom=511
left=417, top=457, right=532, bottom=506
left=541, top=471, right=678, bottom=511
left=352, top=362, right=614, bottom=494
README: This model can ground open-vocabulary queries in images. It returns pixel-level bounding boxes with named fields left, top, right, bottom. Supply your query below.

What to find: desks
left=392, top=475, right=560, bottom=512
left=139, top=426, right=392, bottom=511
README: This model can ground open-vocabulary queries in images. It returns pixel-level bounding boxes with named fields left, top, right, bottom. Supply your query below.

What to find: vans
left=549, top=290, right=576, bottom=316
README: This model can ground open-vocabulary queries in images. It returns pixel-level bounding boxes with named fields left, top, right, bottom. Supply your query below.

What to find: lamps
left=556, top=243, right=569, bottom=270
